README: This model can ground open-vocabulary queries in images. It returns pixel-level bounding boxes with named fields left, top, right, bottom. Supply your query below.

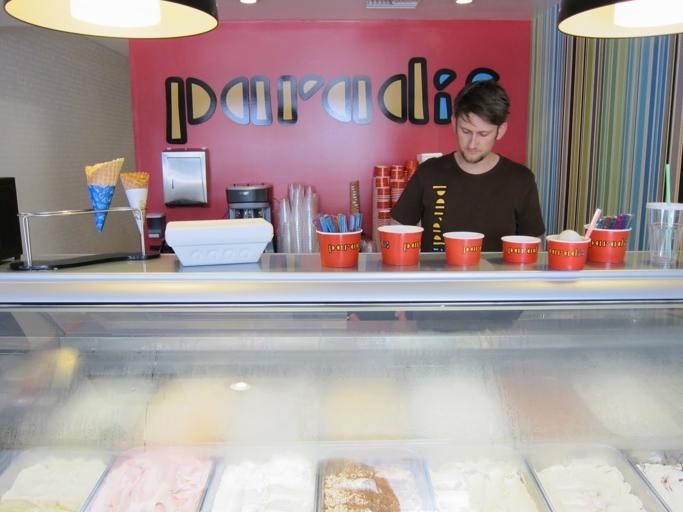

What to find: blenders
left=146, top=214, right=165, bottom=254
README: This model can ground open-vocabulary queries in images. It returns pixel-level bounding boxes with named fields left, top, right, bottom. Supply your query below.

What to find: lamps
left=3, top=0, right=220, bottom=39
left=554, top=0, right=683, bottom=40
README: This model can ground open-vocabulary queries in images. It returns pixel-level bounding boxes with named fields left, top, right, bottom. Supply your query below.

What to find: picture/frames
left=161, top=151, right=207, bottom=207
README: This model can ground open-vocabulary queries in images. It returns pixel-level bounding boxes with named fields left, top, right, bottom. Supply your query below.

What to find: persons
left=387, top=79, right=545, bottom=252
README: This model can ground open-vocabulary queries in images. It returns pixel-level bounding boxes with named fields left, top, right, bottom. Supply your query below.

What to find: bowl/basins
left=502, top=235, right=542, bottom=265
left=374, top=159, right=418, bottom=219
left=442, top=231, right=484, bottom=266
left=378, top=225, right=424, bottom=267
left=316, top=229, right=362, bottom=268
left=583, top=223, right=632, bottom=265
left=545, top=235, right=590, bottom=272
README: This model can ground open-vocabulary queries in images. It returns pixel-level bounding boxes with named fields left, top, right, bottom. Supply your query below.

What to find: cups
left=278, top=182, right=319, bottom=252
left=645, top=203, right=683, bottom=267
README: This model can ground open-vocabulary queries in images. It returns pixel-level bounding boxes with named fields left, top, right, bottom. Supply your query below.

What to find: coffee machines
left=226, top=185, right=273, bottom=252
